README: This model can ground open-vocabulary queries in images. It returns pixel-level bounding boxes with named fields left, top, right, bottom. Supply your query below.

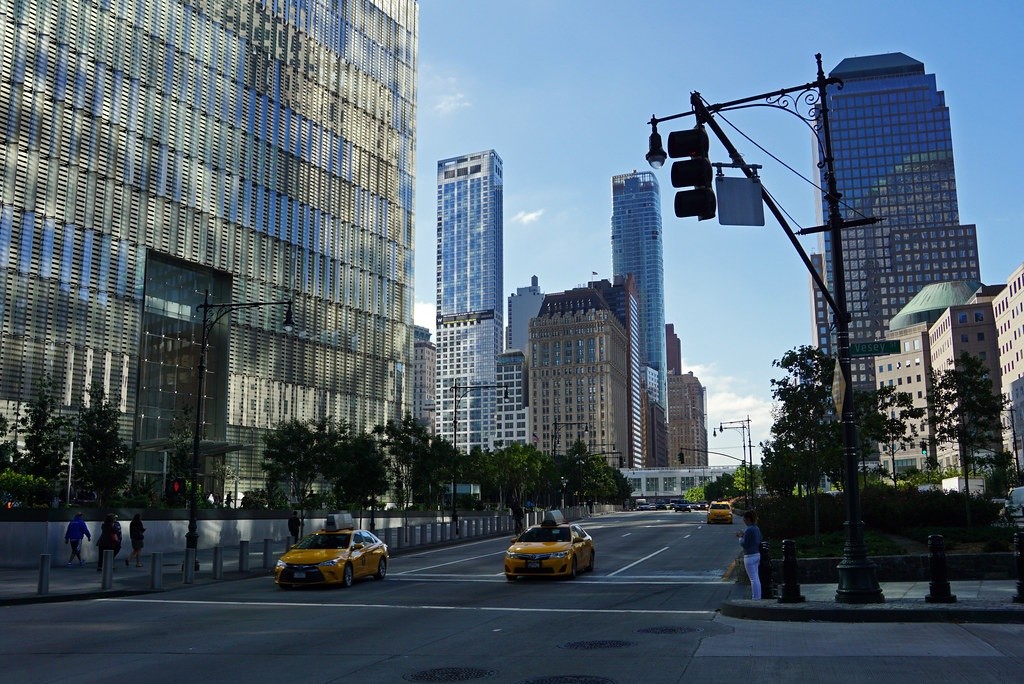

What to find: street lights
left=452, top=381, right=511, bottom=533
left=648, top=87, right=883, bottom=608
left=588, top=443, right=616, bottom=455
left=713, top=421, right=756, bottom=509
left=182, top=299, right=295, bottom=569
left=553, top=422, right=590, bottom=508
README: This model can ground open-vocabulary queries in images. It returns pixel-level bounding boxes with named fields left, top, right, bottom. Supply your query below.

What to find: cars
left=503, top=510, right=595, bottom=580
left=690, top=501, right=708, bottom=510
left=1005, top=487, right=1024, bottom=530
left=646, top=502, right=670, bottom=510
left=274, top=514, right=390, bottom=589
left=675, top=500, right=691, bottom=512
left=706, top=501, right=734, bottom=524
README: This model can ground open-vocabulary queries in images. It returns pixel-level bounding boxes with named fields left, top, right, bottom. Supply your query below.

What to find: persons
left=64, top=512, right=91, bottom=565
left=225, top=491, right=233, bottom=506
left=96, top=513, right=122, bottom=571
left=512, top=501, right=524, bottom=533
left=288, top=511, right=301, bottom=544
left=125, top=512, right=146, bottom=567
left=736, top=510, right=761, bottom=600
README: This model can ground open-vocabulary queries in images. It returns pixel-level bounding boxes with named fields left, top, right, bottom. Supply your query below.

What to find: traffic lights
left=174, top=480, right=186, bottom=508
left=666, top=126, right=715, bottom=218
left=678, top=453, right=685, bottom=464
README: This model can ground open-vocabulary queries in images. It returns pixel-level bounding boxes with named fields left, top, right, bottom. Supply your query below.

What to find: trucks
left=943, top=475, right=985, bottom=495
left=670, top=499, right=679, bottom=509
left=636, top=499, right=646, bottom=509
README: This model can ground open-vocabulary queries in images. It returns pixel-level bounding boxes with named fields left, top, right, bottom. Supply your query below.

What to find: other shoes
left=125, top=559, right=129, bottom=566
left=96, top=567, right=102, bottom=571
left=80, top=560, right=86, bottom=566
left=68, top=563, right=73, bottom=567
left=136, top=564, right=143, bottom=567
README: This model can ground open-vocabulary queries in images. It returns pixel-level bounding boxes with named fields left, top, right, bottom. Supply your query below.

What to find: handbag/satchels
left=112, top=534, right=118, bottom=543
